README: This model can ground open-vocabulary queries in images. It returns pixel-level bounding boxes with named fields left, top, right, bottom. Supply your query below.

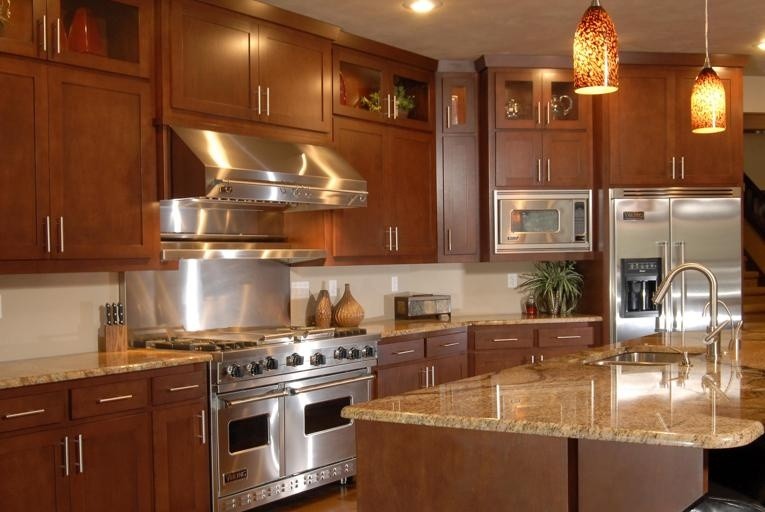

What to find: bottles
left=527, top=291, right=536, bottom=313
left=68, top=6, right=106, bottom=58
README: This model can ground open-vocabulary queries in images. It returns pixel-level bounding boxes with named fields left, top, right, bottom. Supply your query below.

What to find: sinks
left=607, top=345, right=708, bottom=360
left=581, top=355, right=672, bottom=367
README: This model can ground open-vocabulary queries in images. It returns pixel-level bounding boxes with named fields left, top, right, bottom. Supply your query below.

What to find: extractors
left=161, top=127, right=368, bottom=251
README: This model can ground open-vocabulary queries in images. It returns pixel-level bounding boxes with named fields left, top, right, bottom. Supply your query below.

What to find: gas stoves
left=144, top=334, right=261, bottom=352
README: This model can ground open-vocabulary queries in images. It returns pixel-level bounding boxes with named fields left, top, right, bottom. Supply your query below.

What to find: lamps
left=689, top=0, right=726, bottom=134
left=402, top=0, right=444, bottom=12
left=572, top=0, right=619, bottom=95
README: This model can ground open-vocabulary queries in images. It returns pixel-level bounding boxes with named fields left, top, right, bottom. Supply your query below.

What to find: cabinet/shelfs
left=0, top=0, right=155, bottom=79
left=285, top=115, right=435, bottom=258
left=0, top=54, right=154, bottom=261
left=376, top=324, right=594, bottom=401
left=330, top=43, right=435, bottom=135
left=151, top=367, right=213, bottom=512
left=168, top=0, right=332, bottom=135
left=435, top=68, right=742, bottom=261
left=0, top=377, right=154, bottom=512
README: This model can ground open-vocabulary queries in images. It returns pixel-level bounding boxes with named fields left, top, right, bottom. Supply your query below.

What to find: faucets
left=652, top=262, right=721, bottom=359
left=652, top=363, right=720, bottom=438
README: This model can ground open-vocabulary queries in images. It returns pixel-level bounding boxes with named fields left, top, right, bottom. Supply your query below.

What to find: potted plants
left=516, top=258, right=585, bottom=315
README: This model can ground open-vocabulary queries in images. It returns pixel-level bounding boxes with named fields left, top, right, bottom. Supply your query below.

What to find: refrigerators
left=607, top=187, right=742, bottom=346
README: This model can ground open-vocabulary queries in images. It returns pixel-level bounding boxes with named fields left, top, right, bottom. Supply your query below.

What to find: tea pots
left=549, top=94, right=573, bottom=122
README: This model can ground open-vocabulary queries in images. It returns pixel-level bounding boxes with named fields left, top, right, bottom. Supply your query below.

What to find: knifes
left=104, top=302, right=127, bottom=327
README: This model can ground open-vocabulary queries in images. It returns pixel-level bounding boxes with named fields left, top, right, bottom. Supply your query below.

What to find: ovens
left=211, top=369, right=378, bottom=512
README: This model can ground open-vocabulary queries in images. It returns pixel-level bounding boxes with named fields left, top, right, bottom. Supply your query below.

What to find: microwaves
left=494, top=188, right=595, bottom=254
